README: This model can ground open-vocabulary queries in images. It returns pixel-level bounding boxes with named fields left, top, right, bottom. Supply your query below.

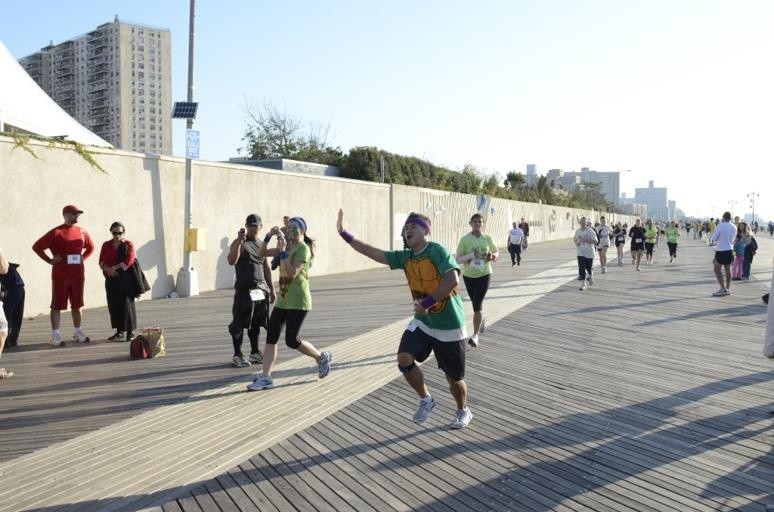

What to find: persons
left=247, top=217, right=332, bottom=391
left=0, top=248, right=15, bottom=379
left=336, top=209, right=474, bottom=429
left=454, top=213, right=499, bottom=346
left=277, top=216, right=290, bottom=247
left=2, top=262, right=25, bottom=347
left=508, top=222, right=524, bottom=267
left=31, top=205, right=95, bottom=346
left=573, top=212, right=774, bottom=296
left=518, top=217, right=529, bottom=249
left=98, top=222, right=138, bottom=342
left=227, top=214, right=275, bottom=368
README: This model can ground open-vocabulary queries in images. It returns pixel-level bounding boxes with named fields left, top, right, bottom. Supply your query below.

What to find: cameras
left=276, top=229, right=285, bottom=240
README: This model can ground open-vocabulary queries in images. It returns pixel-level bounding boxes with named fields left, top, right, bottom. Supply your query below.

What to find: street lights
left=728, top=200, right=738, bottom=221
left=748, top=191, right=761, bottom=225
left=612, top=170, right=632, bottom=225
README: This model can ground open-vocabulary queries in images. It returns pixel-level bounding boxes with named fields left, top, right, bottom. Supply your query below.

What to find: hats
left=246, top=214, right=262, bottom=227
left=63, top=205, right=84, bottom=214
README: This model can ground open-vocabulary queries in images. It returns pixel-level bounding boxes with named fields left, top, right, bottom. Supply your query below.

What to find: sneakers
left=577, top=254, right=677, bottom=292
left=232, top=354, right=252, bottom=368
left=731, top=275, right=750, bottom=280
left=316, top=351, right=332, bottom=379
left=512, top=264, right=521, bottom=269
left=71, top=331, right=89, bottom=343
left=712, top=288, right=730, bottom=296
left=49, top=334, right=66, bottom=346
left=413, top=396, right=437, bottom=422
left=467, top=316, right=488, bottom=348
left=0, top=368, right=14, bottom=379
left=247, top=375, right=274, bottom=391
left=108, top=331, right=136, bottom=342
left=249, top=351, right=265, bottom=364
left=449, top=406, right=473, bottom=429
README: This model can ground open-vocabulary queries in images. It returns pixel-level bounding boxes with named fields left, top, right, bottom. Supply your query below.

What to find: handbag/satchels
left=129, top=325, right=166, bottom=361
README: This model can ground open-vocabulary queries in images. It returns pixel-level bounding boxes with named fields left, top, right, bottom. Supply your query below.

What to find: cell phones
left=240, top=228, right=245, bottom=236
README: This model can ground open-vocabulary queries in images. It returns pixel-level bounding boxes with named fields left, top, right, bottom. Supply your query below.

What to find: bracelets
left=339, top=231, right=353, bottom=242
left=421, top=295, right=436, bottom=309
left=112, top=266, right=117, bottom=271
left=264, top=234, right=270, bottom=242
left=280, top=252, right=288, bottom=259
